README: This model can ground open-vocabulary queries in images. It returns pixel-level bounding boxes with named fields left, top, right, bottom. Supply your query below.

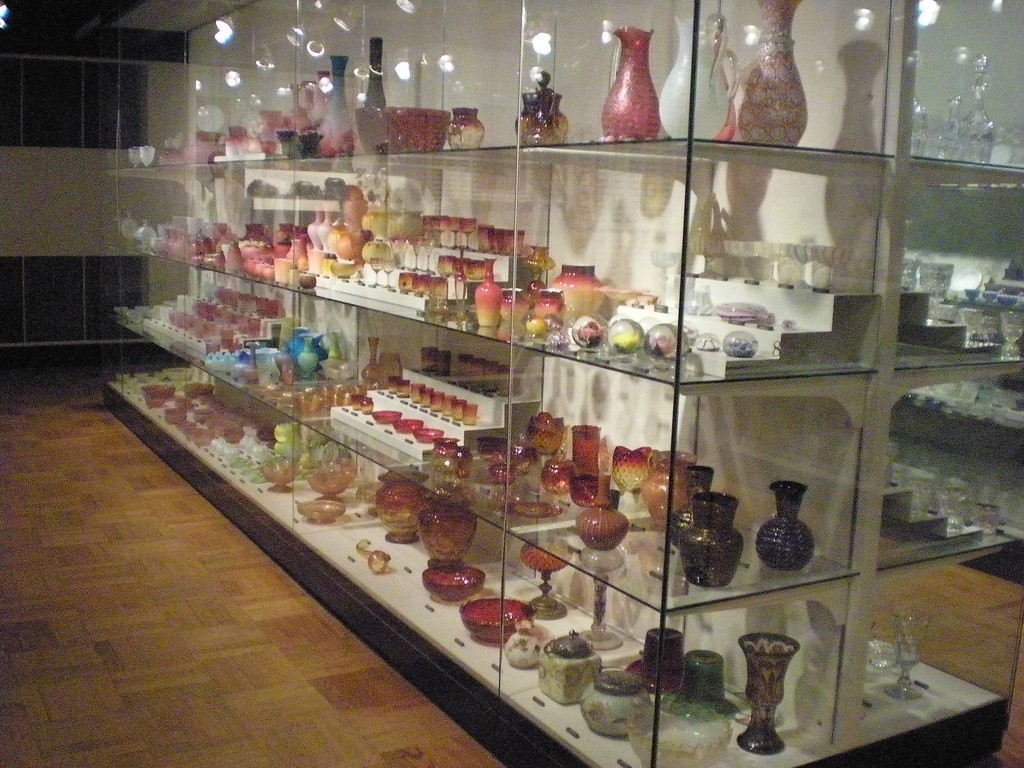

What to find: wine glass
left=958, top=307, right=1024, bottom=362
left=128, top=144, right=155, bottom=167
left=901, top=256, right=954, bottom=326
left=885, top=608, right=928, bottom=700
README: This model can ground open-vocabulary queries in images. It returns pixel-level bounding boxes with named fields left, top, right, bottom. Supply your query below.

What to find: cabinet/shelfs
left=102, top=131, right=904, bottom=768
left=850, top=156, right=1024, bottom=768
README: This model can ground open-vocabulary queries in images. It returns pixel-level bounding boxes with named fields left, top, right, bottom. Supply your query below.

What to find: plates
left=949, top=266, right=982, bottom=292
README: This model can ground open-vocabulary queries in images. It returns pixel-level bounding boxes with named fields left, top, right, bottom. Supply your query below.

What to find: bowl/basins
left=299, top=498, right=347, bottom=524
left=370, top=409, right=445, bottom=442
left=459, top=597, right=538, bottom=645
left=258, top=464, right=300, bottom=493
left=354, top=104, right=451, bottom=154
left=422, top=565, right=487, bottom=607
left=476, top=434, right=507, bottom=461
left=305, top=474, right=352, bottom=502
left=964, top=289, right=1017, bottom=308
left=627, top=702, right=732, bottom=767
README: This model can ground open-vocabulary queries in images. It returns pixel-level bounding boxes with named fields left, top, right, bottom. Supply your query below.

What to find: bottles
left=913, top=55, right=994, bottom=163
left=447, top=106, right=485, bottom=150
left=288, top=327, right=326, bottom=373
left=361, top=335, right=382, bottom=383
left=473, top=256, right=605, bottom=329
left=576, top=474, right=629, bottom=552
left=516, top=91, right=568, bottom=147
left=308, top=209, right=334, bottom=250
left=138, top=219, right=155, bottom=244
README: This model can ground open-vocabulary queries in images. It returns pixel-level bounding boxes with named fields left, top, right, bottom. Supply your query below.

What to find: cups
left=527, top=410, right=562, bottom=455
left=539, top=459, right=578, bottom=494
left=570, top=423, right=601, bottom=475
left=613, top=444, right=656, bottom=494
left=148, top=236, right=166, bottom=253
left=273, top=257, right=292, bottom=284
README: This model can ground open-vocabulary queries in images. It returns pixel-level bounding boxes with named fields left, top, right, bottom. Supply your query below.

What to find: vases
left=113, top=1, right=817, bottom=768
